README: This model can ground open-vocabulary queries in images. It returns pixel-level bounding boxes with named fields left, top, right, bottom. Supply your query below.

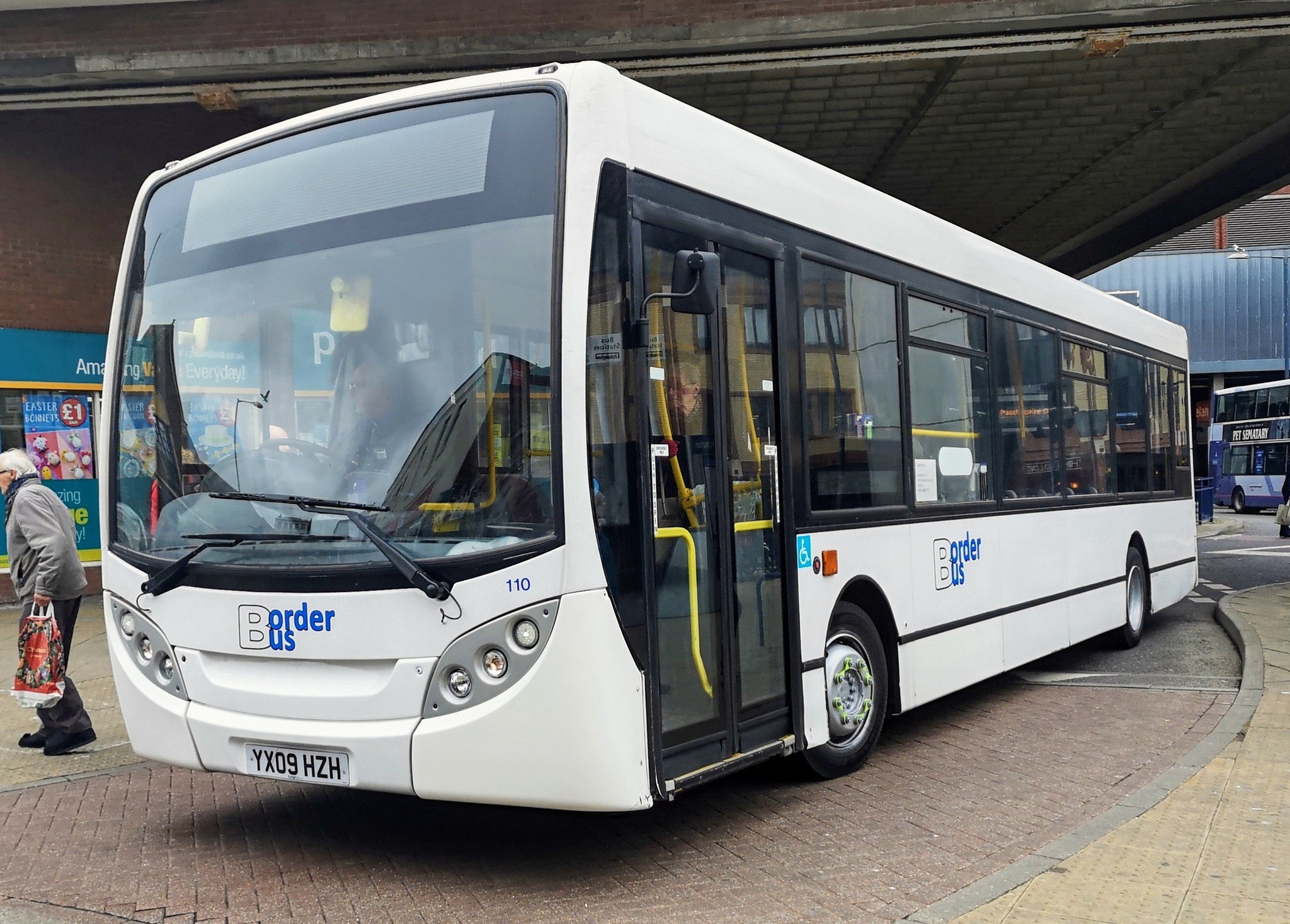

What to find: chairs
left=472, top=473, right=546, bottom=523
left=404, top=357, right=480, bottom=503
left=1217, top=399, right=1290, bottom=424
left=1233, top=462, right=1246, bottom=474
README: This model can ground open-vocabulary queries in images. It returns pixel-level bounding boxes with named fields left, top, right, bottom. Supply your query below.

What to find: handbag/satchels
left=1275, top=499, right=1290, bottom=525
left=11, top=601, right=65, bottom=708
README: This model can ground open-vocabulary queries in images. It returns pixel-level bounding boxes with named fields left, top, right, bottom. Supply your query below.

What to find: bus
left=99, top=59, right=1198, bottom=814
left=1209, top=379, right=1290, bottom=514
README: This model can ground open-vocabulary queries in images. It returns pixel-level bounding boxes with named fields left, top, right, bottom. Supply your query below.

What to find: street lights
left=233, top=397, right=263, bottom=492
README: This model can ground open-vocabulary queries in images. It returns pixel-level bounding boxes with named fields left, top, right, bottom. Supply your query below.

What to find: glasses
left=349, top=383, right=375, bottom=392
left=664, top=382, right=697, bottom=392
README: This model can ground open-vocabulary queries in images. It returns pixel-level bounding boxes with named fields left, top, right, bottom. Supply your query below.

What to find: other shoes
left=1279, top=531, right=1290, bottom=538
left=43, top=727, right=97, bottom=756
left=18, top=733, right=46, bottom=748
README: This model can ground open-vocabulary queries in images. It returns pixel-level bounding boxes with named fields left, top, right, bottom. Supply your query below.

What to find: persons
left=0, top=448, right=96, bottom=756
left=334, top=362, right=414, bottom=476
left=1279, top=467, right=1290, bottom=538
left=658, top=362, right=712, bottom=498
